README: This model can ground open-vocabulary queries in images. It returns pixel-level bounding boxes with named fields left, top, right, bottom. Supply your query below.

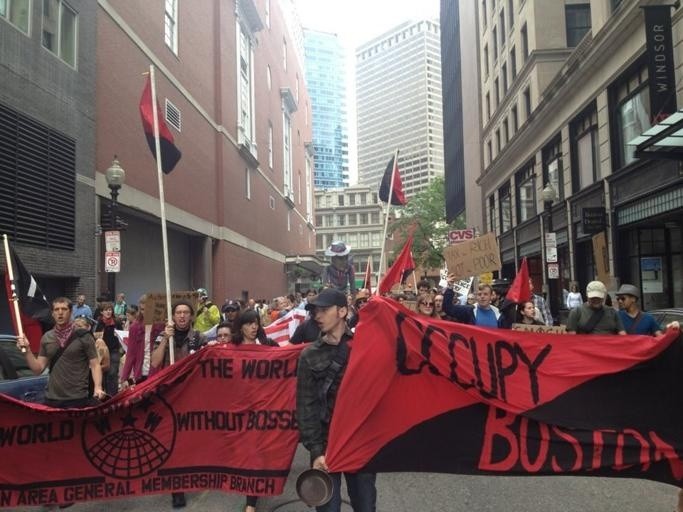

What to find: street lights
left=103, top=152, right=126, bottom=304
left=540, top=181, right=564, bottom=330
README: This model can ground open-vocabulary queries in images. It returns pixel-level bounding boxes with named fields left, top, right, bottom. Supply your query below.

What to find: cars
left=0, top=333, right=50, bottom=408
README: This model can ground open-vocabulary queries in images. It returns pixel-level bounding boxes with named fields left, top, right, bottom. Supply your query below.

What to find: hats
left=324, top=242, right=352, bottom=257
left=309, top=288, right=347, bottom=308
left=354, top=289, right=369, bottom=302
left=586, top=280, right=605, bottom=298
left=615, top=284, right=641, bottom=299
left=221, top=299, right=240, bottom=313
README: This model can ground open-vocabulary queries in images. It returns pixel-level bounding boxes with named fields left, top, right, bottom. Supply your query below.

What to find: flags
left=137, top=75, right=181, bottom=176
left=378, top=156, right=404, bottom=205
left=3, top=245, right=55, bottom=350
left=377, top=238, right=415, bottom=293
left=364, top=264, right=372, bottom=288
left=506, top=260, right=531, bottom=303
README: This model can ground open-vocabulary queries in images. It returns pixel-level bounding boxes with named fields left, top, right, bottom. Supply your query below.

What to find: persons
left=296, top=291, right=377, bottom=512
left=1, top=288, right=371, bottom=387
left=233, top=313, right=280, bottom=512
left=152, top=301, right=204, bottom=508
left=382, top=280, right=680, bottom=336
left=324, top=242, right=355, bottom=292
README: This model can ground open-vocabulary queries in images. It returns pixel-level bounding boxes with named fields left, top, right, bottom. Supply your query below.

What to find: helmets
left=197, top=287, right=209, bottom=298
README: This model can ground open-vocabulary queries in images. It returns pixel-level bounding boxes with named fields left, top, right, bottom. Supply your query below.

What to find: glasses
left=420, top=302, right=434, bottom=307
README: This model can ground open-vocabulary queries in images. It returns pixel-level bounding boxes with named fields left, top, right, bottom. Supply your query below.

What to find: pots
left=294, top=465, right=334, bottom=506
